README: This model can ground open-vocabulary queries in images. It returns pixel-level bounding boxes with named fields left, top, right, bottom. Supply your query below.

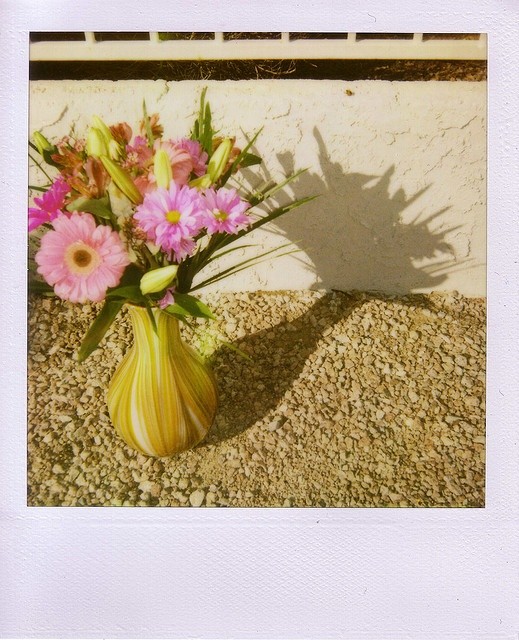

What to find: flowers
left=28, top=84, right=320, bottom=366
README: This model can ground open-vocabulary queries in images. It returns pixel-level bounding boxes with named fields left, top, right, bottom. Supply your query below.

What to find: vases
left=103, top=302, right=220, bottom=460
